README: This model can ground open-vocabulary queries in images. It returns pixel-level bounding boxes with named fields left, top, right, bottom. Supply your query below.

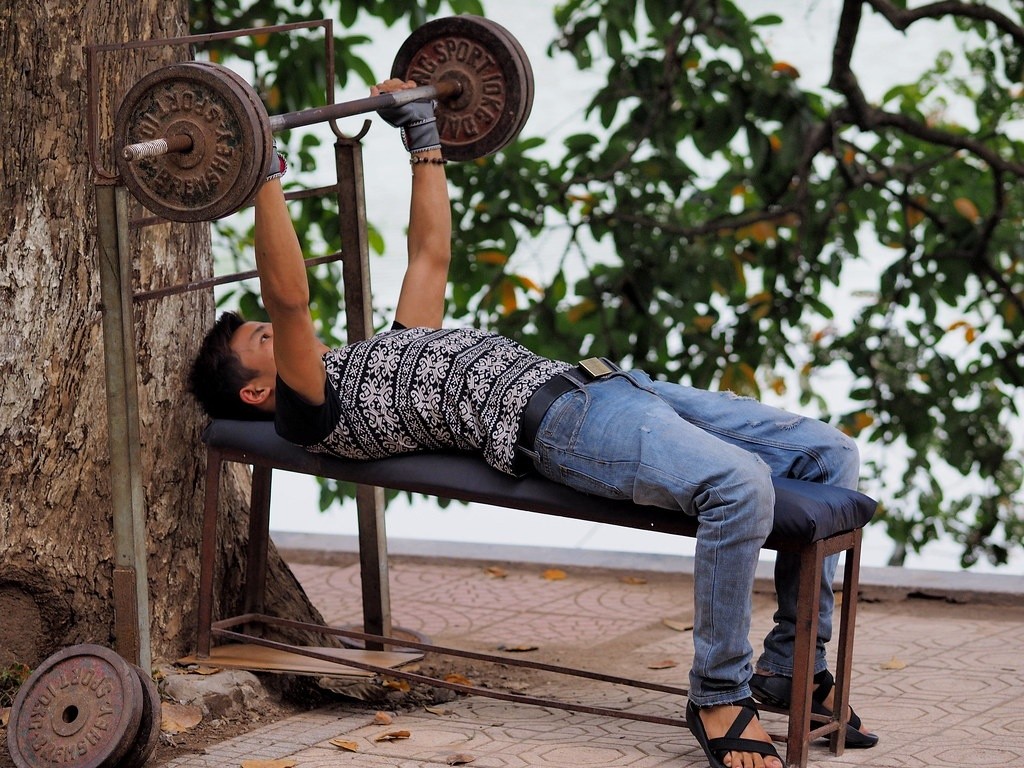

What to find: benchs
left=196, top=419, right=878, bottom=768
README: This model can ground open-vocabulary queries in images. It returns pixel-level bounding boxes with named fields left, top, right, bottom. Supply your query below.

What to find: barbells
left=111, top=15, right=536, bottom=224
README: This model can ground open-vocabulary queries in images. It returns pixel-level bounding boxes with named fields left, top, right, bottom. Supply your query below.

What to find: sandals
left=750, top=664, right=878, bottom=750
left=686, top=688, right=786, bottom=768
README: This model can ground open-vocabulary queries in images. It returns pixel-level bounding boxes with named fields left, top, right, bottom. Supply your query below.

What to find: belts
left=516, top=357, right=617, bottom=473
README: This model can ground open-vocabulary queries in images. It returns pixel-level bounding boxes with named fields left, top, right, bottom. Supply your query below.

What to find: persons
left=187, top=79, right=880, bottom=768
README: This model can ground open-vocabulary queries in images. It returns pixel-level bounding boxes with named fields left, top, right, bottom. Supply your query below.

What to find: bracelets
left=410, top=155, right=447, bottom=165
